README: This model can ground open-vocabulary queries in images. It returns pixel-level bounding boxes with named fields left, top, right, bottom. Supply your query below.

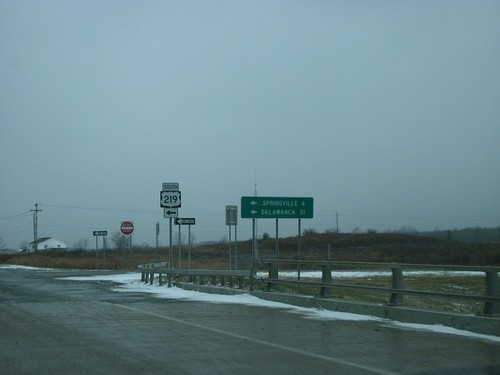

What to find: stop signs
left=119, top=221, right=135, bottom=235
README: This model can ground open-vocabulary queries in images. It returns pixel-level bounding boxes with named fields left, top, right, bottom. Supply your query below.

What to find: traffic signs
left=93, top=231, right=107, bottom=236
left=241, top=196, right=314, bottom=218
left=174, top=218, right=195, bottom=225
